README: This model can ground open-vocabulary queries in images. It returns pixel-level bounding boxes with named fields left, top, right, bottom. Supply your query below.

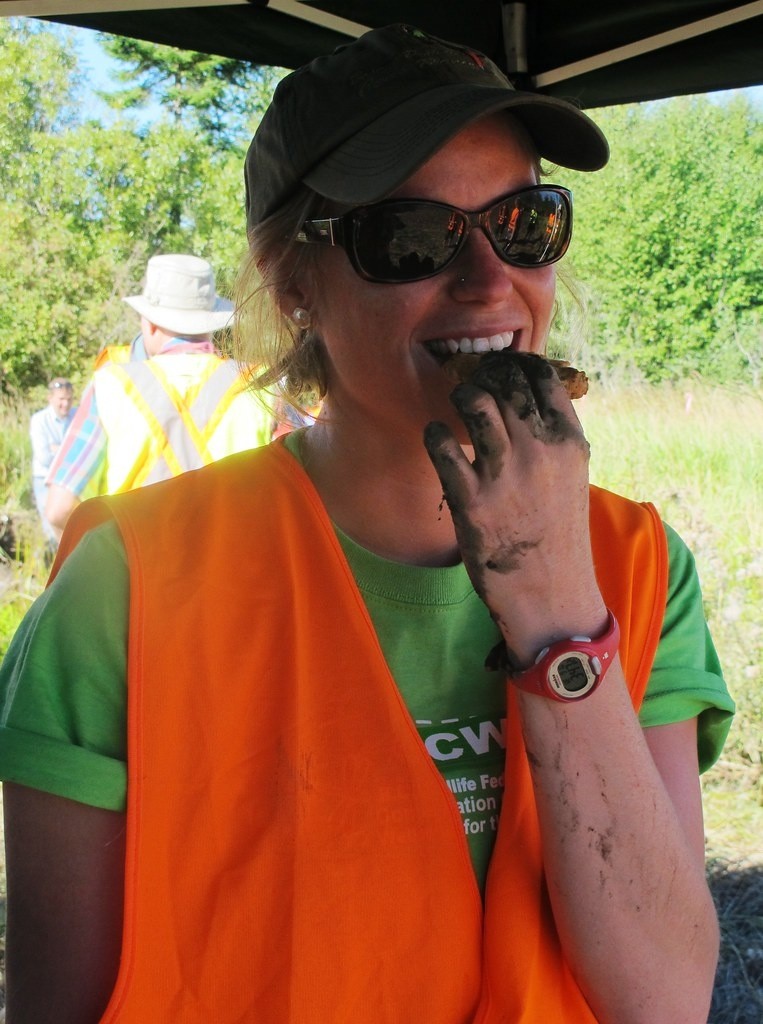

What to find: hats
left=121, top=253, right=235, bottom=335
left=243, top=25, right=611, bottom=247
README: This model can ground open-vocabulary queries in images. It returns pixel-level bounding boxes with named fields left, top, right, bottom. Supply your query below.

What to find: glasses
left=52, top=381, right=74, bottom=389
left=293, top=183, right=575, bottom=285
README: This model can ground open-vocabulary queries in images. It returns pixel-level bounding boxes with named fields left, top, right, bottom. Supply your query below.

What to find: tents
left=4, top=0, right=763, bottom=152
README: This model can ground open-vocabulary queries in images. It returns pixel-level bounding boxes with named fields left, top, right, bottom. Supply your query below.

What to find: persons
left=29, top=376, right=84, bottom=551
left=4, top=28, right=744, bottom=1024
left=35, top=247, right=306, bottom=537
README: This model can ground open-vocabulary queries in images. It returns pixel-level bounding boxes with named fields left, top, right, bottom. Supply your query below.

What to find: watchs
left=486, top=610, right=623, bottom=706
